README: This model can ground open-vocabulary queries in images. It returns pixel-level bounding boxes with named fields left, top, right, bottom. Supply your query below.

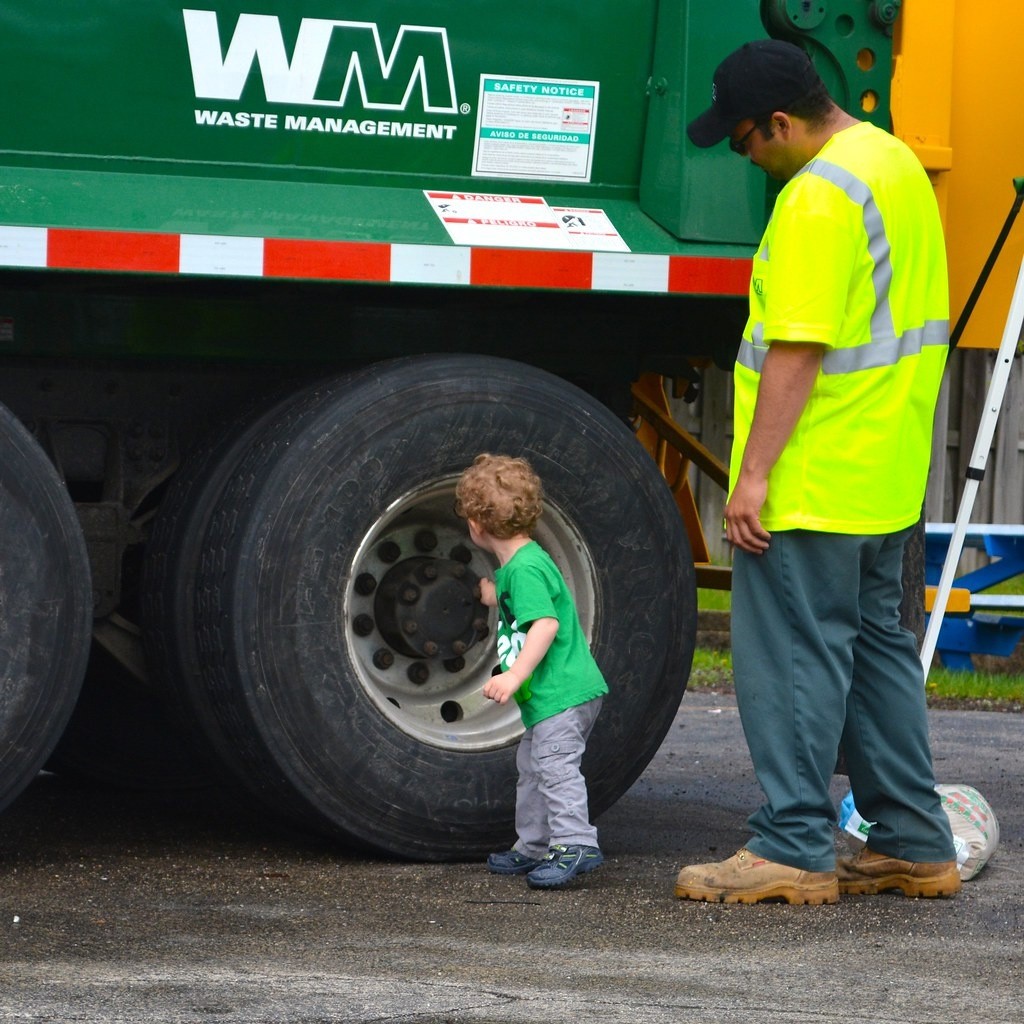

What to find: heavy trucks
left=1, top=0, right=913, bottom=863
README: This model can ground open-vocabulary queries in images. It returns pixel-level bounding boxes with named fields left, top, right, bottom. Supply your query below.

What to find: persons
left=453, top=449, right=609, bottom=886
left=675, top=38, right=965, bottom=907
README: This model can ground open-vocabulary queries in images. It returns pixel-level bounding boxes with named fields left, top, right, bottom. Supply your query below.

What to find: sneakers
left=834, top=844, right=962, bottom=901
left=487, top=842, right=544, bottom=875
left=677, top=838, right=840, bottom=907
left=527, top=835, right=604, bottom=889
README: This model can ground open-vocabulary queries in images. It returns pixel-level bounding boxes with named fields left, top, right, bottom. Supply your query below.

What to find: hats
left=687, top=40, right=819, bottom=151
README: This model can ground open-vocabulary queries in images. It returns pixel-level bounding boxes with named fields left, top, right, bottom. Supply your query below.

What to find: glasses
left=731, top=111, right=772, bottom=155
